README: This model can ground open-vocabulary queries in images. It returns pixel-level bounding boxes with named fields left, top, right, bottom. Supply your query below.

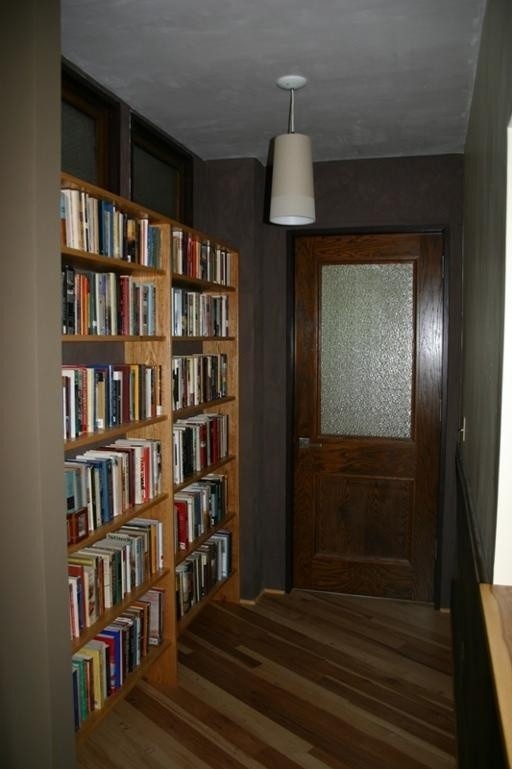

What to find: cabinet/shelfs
left=59, top=168, right=241, bottom=753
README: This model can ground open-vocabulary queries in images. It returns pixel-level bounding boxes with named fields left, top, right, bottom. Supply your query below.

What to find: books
left=177, top=532, right=230, bottom=624
left=62, top=361, right=162, bottom=442
left=174, top=471, right=233, bottom=551
left=172, top=412, right=229, bottom=486
left=73, top=582, right=168, bottom=730
left=173, top=226, right=235, bottom=286
left=169, top=282, right=231, bottom=336
left=62, top=265, right=162, bottom=336
left=68, top=516, right=166, bottom=640
left=64, top=438, right=162, bottom=547
left=61, top=182, right=165, bottom=268
left=173, top=351, right=228, bottom=410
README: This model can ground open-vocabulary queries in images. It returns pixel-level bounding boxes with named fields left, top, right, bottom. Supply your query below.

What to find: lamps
left=270, top=74, right=316, bottom=226
